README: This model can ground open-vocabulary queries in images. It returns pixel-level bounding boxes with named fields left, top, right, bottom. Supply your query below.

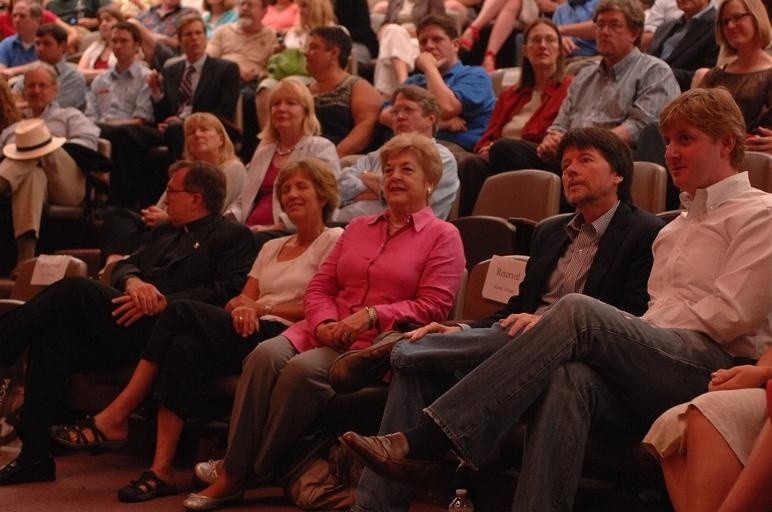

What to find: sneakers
left=194, top=459, right=257, bottom=489
left=183, top=487, right=244, bottom=511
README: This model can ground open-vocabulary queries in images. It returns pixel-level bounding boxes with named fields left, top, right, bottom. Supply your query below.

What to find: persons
left=0, top=0, right=771, bottom=511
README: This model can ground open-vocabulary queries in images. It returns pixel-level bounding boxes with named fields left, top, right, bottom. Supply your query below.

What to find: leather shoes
left=338, top=431, right=444, bottom=486
left=328, top=330, right=405, bottom=395
left=0, top=453, right=56, bottom=485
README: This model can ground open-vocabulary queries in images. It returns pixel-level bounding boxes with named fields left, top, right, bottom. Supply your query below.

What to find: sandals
left=460, top=24, right=481, bottom=52
left=482, top=52, right=496, bottom=72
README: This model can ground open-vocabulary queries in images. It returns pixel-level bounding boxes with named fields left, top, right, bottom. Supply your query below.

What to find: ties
left=175, top=65, right=196, bottom=114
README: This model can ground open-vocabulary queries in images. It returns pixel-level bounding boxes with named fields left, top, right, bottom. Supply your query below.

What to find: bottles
left=275, top=33, right=285, bottom=49
left=449, top=489, right=473, bottom=512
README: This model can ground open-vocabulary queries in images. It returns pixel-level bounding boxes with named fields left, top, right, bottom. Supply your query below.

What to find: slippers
left=50, top=412, right=129, bottom=451
left=118, top=471, right=179, bottom=502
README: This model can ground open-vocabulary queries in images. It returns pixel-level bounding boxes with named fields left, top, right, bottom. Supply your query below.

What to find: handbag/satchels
left=284, top=433, right=364, bottom=511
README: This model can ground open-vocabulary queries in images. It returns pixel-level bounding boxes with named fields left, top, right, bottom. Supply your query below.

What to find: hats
left=2, top=118, right=67, bottom=160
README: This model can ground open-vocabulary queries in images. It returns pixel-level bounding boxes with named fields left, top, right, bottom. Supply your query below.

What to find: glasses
left=717, top=13, right=751, bottom=27
left=166, top=186, right=198, bottom=197
